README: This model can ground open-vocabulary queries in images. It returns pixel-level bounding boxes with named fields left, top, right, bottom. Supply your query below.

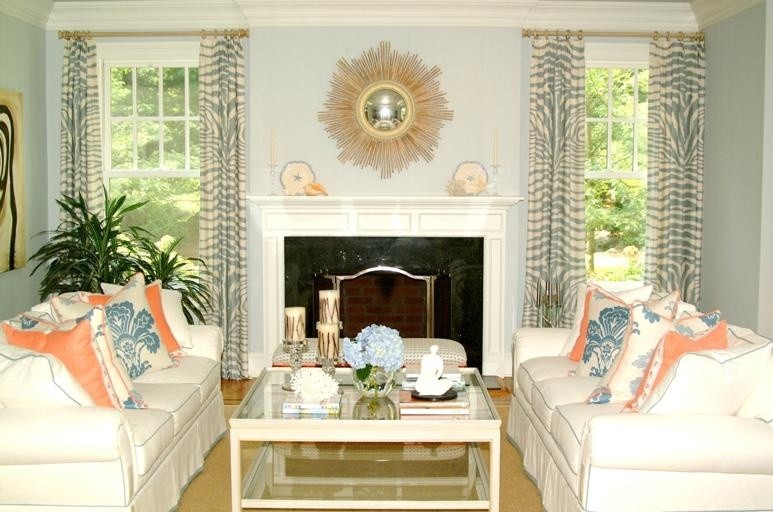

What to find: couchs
left=511, top=285, right=773, bottom=512
left=0, top=286, right=225, bottom=512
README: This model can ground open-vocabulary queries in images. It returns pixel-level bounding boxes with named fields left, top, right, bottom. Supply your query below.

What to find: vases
left=351, top=363, right=397, bottom=397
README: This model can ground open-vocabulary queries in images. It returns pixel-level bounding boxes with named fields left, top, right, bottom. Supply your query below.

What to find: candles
left=494, top=133, right=497, bottom=166
left=270, top=127, right=276, bottom=164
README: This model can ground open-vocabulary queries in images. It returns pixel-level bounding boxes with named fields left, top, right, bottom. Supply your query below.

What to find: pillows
left=2, top=271, right=194, bottom=410
left=560, top=278, right=773, bottom=417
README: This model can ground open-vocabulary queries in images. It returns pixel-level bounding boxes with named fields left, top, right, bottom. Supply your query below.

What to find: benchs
left=275, top=333, right=468, bottom=372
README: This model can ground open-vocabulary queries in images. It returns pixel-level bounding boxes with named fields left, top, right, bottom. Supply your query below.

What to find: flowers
left=341, top=323, right=408, bottom=399
